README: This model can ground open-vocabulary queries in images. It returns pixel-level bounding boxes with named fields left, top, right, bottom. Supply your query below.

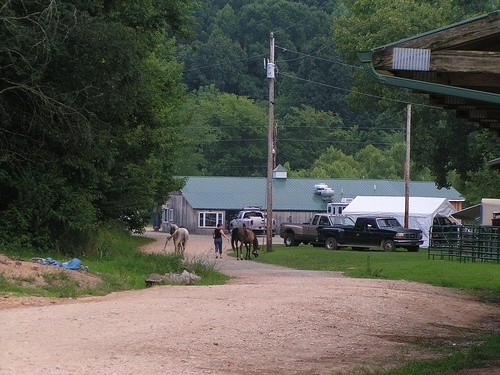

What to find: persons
left=213, top=224, right=230, bottom=258
left=170, top=223, right=179, bottom=245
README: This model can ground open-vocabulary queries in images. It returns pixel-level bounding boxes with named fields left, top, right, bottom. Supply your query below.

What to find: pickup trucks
left=230, top=210, right=276, bottom=237
left=317, top=216, right=423, bottom=252
left=280, top=214, right=356, bottom=246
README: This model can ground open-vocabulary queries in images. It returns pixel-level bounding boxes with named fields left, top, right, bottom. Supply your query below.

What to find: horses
left=163, top=222, right=189, bottom=255
left=231, top=227, right=261, bottom=261
